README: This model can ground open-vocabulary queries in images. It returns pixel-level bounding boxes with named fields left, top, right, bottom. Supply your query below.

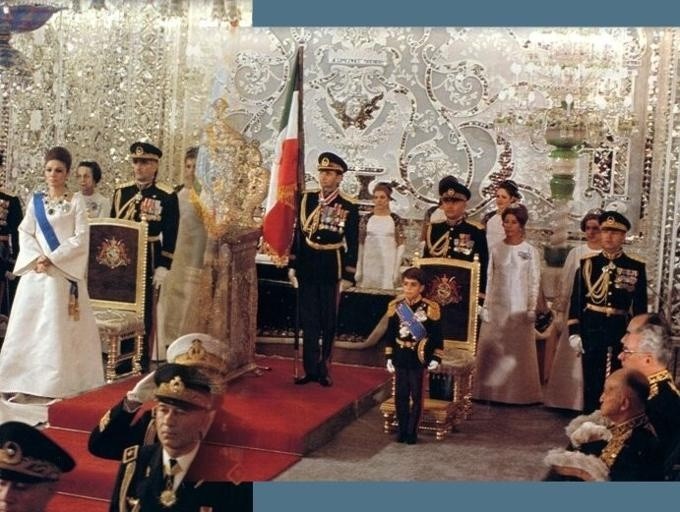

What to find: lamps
left=498, top=27, right=649, bottom=267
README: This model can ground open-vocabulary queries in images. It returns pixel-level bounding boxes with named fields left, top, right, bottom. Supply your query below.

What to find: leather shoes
left=317, top=374, right=332, bottom=386
left=398, top=433, right=407, bottom=442
left=407, top=433, right=417, bottom=444
left=294, top=375, right=317, bottom=384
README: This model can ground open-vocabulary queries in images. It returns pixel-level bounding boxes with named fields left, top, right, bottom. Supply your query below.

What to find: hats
left=0, top=421, right=76, bottom=483
left=130, top=142, right=162, bottom=161
left=440, top=181, right=471, bottom=201
left=154, top=363, right=212, bottom=412
left=318, top=152, right=347, bottom=174
left=166, top=333, right=232, bottom=377
left=599, top=211, right=630, bottom=233
left=417, top=338, right=431, bottom=368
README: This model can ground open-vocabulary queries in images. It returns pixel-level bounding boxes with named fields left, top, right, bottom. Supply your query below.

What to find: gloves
left=427, top=360, right=439, bottom=369
left=338, top=279, right=354, bottom=296
left=386, top=359, right=396, bottom=373
left=288, top=269, right=299, bottom=288
left=152, top=266, right=168, bottom=289
left=569, top=336, right=585, bottom=355
left=127, top=371, right=156, bottom=403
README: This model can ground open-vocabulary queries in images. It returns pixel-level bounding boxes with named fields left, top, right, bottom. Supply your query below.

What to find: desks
left=536, top=266, right=563, bottom=383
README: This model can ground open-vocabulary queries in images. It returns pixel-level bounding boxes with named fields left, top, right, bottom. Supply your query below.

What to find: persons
left=107, top=360, right=251, bottom=511
left=385, top=267, right=442, bottom=444
left=0, top=143, right=607, bottom=412
left=1, top=420, right=76, bottom=509
left=567, top=323, right=679, bottom=479
left=618, top=312, right=672, bottom=347
left=88, top=330, right=251, bottom=482
left=538, top=369, right=663, bottom=482
left=563, top=212, right=649, bottom=414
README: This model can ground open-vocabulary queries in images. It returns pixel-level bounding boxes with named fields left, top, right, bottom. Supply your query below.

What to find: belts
left=147, top=236, right=160, bottom=242
left=395, top=338, right=416, bottom=347
left=305, top=237, right=344, bottom=250
left=587, top=303, right=624, bottom=314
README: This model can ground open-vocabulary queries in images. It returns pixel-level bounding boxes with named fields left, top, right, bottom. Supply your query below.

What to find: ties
left=164, top=459, right=177, bottom=491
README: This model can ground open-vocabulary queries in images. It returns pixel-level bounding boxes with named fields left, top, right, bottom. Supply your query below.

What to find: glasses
left=623, top=349, right=651, bottom=358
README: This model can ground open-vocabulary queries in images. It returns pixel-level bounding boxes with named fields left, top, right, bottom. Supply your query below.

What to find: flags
left=262, top=49, right=301, bottom=263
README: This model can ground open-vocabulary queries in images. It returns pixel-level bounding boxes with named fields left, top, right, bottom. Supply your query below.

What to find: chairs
left=376, top=247, right=483, bottom=442
left=72, top=207, right=153, bottom=385
